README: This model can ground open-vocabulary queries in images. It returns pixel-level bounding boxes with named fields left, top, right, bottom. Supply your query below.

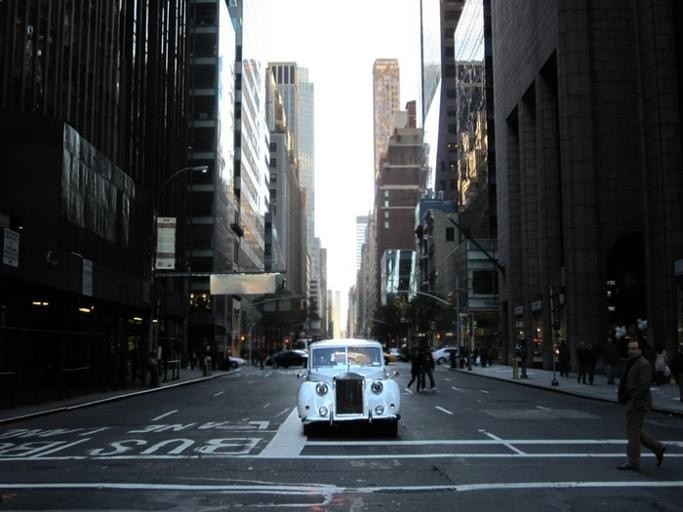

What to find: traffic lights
left=394, top=296, right=408, bottom=309
left=447, top=292, right=456, bottom=307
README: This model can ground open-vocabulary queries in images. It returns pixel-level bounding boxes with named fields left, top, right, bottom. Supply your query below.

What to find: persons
left=615, top=338, right=667, bottom=471
left=582, top=340, right=602, bottom=385
left=472, top=347, right=478, bottom=367
left=669, top=348, right=683, bottom=402
left=486, top=346, right=494, bottom=366
left=405, top=349, right=423, bottom=393
left=421, top=344, right=437, bottom=392
left=575, top=343, right=587, bottom=384
left=186, top=352, right=199, bottom=378
left=478, top=347, right=488, bottom=367
left=558, top=338, right=570, bottom=378
left=651, top=341, right=668, bottom=385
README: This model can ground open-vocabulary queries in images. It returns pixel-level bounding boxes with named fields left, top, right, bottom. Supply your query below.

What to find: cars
left=228, top=356, right=245, bottom=367
left=296, top=338, right=401, bottom=441
left=272, top=349, right=308, bottom=368
left=430, top=348, right=459, bottom=365
left=384, top=351, right=400, bottom=364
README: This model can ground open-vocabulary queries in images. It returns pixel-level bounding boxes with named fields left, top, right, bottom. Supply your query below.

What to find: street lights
left=157, top=165, right=209, bottom=226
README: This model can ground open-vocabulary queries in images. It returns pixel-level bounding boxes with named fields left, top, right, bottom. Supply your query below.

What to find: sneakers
left=577, top=380, right=616, bottom=385
left=615, top=460, right=640, bottom=471
left=405, top=385, right=437, bottom=393
left=653, top=444, right=666, bottom=468
